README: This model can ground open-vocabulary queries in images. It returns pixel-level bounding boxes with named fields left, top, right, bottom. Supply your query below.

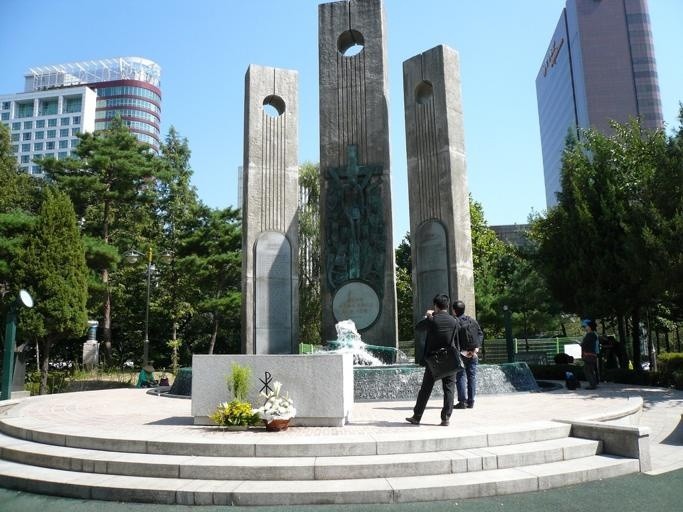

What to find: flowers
left=208, top=362, right=260, bottom=427
left=253, top=381, right=296, bottom=425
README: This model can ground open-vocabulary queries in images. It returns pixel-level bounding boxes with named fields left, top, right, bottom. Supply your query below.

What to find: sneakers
left=453, top=400, right=466, bottom=409
left=440, top=416, right=450, bottom=427
left=584, top=385, right=597, bottom=390
left=405, top=416, right=419, bottom=426
left=466, top=400, right=475, bottom=408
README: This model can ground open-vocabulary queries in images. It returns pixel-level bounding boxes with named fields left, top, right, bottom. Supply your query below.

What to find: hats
left=587, top=321, right=597, bottom=331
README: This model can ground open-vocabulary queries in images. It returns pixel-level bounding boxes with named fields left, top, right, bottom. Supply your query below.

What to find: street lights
left=120, top=246, right=177, bottom=366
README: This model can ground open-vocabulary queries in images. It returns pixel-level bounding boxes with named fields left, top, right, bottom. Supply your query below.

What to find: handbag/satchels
left=423, top=345, right=465, bottom=380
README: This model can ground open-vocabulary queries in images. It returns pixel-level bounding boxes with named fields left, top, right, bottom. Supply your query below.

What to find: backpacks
left=455, top=316, right=479, bottom=352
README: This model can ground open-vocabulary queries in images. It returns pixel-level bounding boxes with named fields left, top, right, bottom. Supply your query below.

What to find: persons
left=578, top=321, right=597, bottom=389
left=404, top=293, right=459, bottom=425
left=581, top=319, right=600, bottom=385
left=135, top=365, right=159, bottom=388
left=449, top=300, right=483, bottom=409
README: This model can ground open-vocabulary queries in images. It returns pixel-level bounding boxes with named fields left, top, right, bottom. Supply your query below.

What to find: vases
left=225, top=422, right=249, bottom=431
left=262, top=418, right=289, bottom=431
